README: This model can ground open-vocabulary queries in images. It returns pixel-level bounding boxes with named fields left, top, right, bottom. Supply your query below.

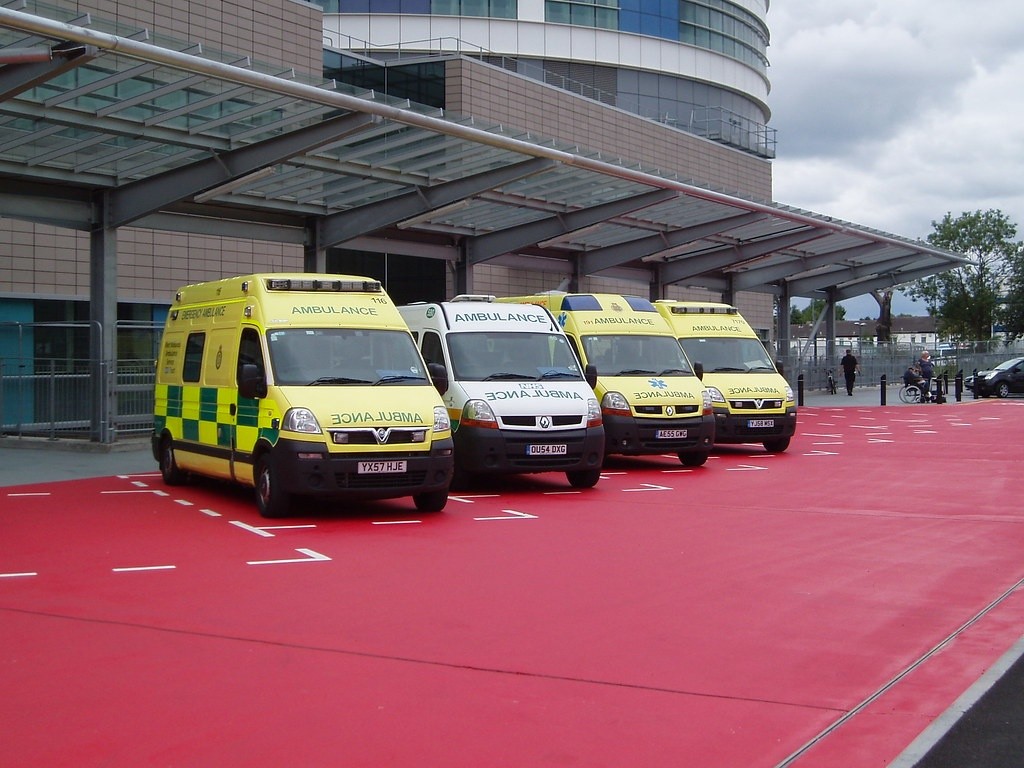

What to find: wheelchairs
left=899, top=375, right=934, bottom=405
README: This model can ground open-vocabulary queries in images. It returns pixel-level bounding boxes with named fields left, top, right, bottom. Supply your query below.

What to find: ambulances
left=371, top=295, right=606, bottom=489
left=548, top=300, right=798, bottom=453
left=487, top=289, right=716, bottom=469
left=151, top=271, right=455, bottom=518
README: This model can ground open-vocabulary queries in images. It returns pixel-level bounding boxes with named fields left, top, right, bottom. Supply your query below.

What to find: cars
left=964, top=356, right=1024, bottom=398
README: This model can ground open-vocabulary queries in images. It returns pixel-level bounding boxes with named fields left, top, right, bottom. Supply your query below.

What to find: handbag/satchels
left=917, top=376, right=925, bottom=384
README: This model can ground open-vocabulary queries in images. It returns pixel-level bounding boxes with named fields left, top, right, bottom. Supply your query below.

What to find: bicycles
left=824, top=367, right=839, bottom=395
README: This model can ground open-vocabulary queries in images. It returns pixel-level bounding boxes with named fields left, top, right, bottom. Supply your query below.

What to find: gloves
left=858, top=370, right=861, bottom=376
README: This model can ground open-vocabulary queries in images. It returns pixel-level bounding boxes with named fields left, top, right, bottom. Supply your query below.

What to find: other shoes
left=916, top=397, right=920, bottom=403
left=847, top=390, right=852, bottom=396
left=927, top=399, right=931, bottom=403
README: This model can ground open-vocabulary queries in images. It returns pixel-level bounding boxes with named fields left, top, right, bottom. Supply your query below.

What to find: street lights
left=809, top=323, right=818, bottom=374
left=854, top=322, right=867, bottom=368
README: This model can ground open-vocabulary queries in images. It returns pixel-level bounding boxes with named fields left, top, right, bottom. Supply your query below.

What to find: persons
left=904, top=351, right=935, bottom=403
left=840, top=349, right=861, bottom=397
